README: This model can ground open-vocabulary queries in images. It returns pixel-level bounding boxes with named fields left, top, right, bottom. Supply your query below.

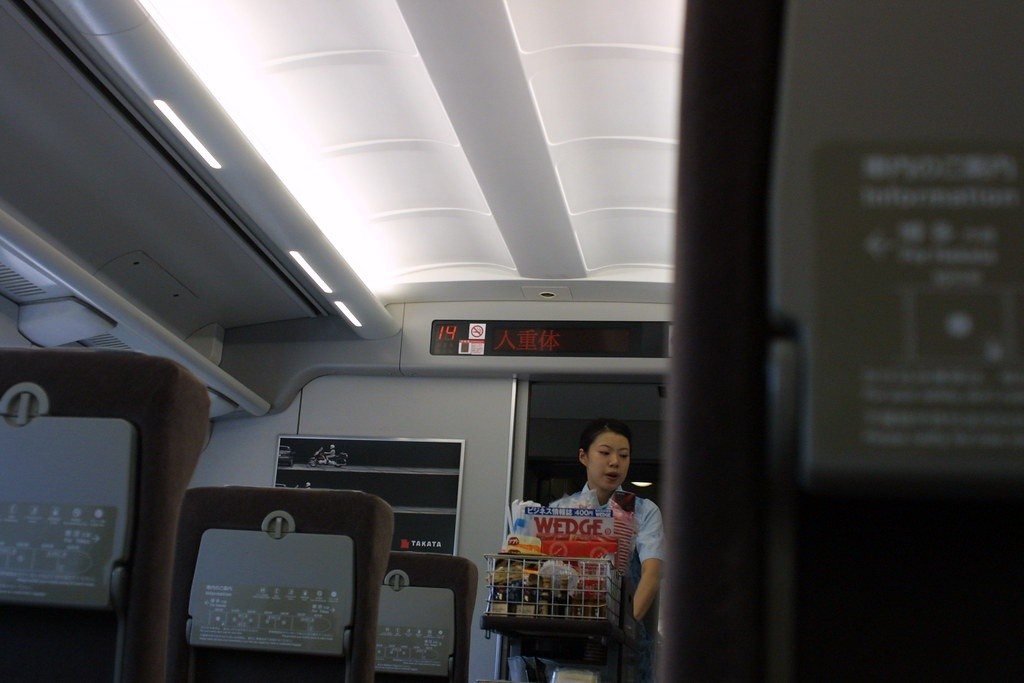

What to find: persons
left=546, top=418, right=664, bottom=683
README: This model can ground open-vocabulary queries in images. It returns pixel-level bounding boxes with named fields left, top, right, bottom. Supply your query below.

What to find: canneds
left=538, top=599, right=606, bottom=622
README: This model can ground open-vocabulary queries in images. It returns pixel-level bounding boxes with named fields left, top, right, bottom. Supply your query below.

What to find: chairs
left=0, top=344, right=212, bottom=683
left=375, top=547, right=480, bottom=683
left=170, top=484, right=395, bottom=683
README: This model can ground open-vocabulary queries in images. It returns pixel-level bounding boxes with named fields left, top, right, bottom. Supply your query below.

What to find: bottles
left=485, top=581, right=605, bottom=619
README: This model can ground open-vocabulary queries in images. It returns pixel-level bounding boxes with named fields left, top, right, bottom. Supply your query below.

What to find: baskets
left=484, top=554, right=624, bottom=627
